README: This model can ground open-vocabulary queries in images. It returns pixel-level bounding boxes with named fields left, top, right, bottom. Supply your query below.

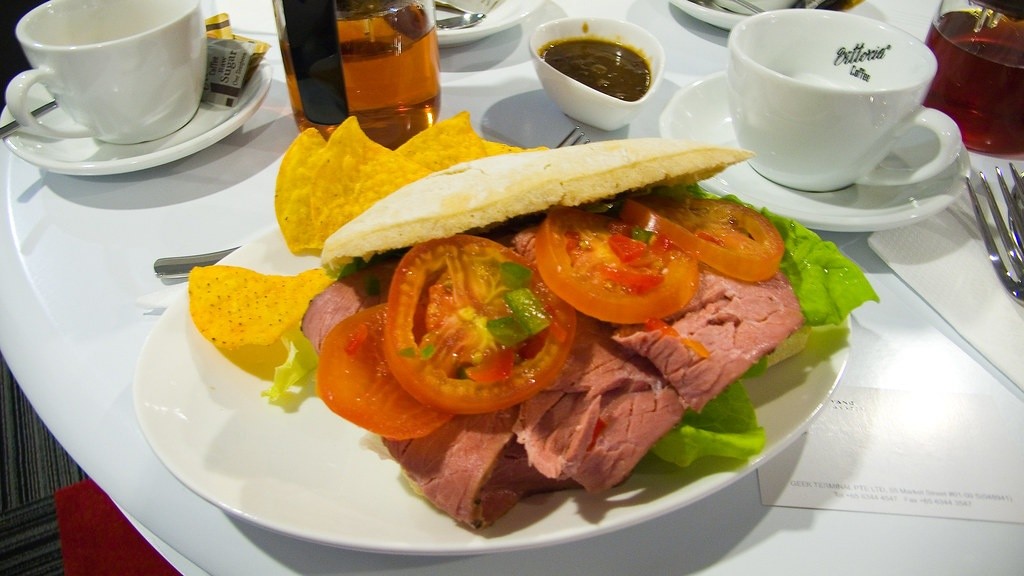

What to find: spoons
left=436, top=13, right=484, bottom=29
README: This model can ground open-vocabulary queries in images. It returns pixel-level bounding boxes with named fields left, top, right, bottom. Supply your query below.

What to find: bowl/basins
left=528, top=16, right=665, bottom=131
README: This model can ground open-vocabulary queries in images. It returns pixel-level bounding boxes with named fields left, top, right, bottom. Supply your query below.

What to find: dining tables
left=0, top=0, right=1024, bottom=576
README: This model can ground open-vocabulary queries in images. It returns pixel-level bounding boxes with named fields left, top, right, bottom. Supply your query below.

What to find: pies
left=322, top=137, right=755, bottom=267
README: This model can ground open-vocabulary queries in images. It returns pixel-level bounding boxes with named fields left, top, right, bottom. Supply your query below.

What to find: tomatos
left=316, top=195, right=785, bottom=440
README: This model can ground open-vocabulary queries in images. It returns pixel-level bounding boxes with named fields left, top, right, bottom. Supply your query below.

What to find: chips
left=190, top=112, right=550, bottom=348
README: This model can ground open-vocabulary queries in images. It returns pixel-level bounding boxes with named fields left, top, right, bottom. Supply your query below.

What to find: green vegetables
left=266, top=327, right=318, bottom=402
left=651, top=185, right=881, bottom=466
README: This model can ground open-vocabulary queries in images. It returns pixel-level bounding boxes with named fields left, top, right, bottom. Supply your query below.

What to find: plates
left=0, top=56, right=274, bottom=176
left=434, top=1, right=544, bottom=49
left=130, top=219, right=855, bottom=556
left=660, top=72, right=971, bottom=233
left=668, top=0, right=750, bottom=31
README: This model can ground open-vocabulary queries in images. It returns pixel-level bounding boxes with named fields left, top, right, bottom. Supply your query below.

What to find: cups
left=5, top=0, right=207, bottom=145
left=725, top=8, right=962, bottom=194
left=271, top=0, right=443, bottom=149
left=922, top=0, right=1024, bottom=162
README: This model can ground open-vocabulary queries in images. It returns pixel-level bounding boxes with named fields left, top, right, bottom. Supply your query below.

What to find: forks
left=966, top=162, right=1024, bottom=305
left=555, top=126, right=590, bottom=149
